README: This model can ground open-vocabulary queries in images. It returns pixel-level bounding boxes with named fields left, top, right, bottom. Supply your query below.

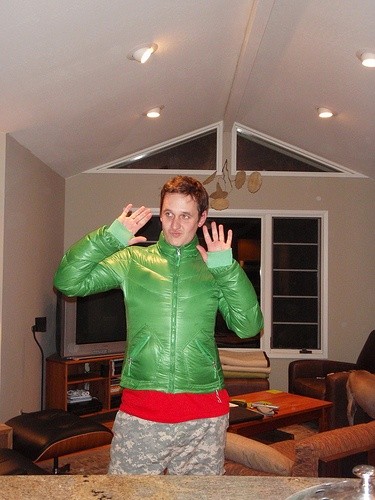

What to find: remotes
left=251, top=403, right=279, bottom=409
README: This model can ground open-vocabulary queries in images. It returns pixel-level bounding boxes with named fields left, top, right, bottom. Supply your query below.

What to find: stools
left=6, top=408, right=113, bottom=464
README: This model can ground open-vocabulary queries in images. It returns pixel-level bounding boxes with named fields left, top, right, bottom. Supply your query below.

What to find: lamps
left=133, top=48, right=152, bottom=64
left=147, top=107, right=161, bottom=118
left=361, top=53, right=375, bottom=68
left=318, top=108, right=333, bottom=118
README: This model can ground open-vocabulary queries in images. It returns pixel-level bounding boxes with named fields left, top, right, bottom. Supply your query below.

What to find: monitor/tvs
left=56, top=287, right=126, bottom=360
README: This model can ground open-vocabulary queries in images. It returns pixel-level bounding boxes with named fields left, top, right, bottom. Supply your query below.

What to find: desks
left=226, top=389, right=335, bottom=434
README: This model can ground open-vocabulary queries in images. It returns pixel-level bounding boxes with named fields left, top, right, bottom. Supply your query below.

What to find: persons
left=52, top=177, right=264, bottom=475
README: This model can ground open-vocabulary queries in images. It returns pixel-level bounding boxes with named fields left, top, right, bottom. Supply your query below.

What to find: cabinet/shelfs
left=45, top=354, right=125, bottom=420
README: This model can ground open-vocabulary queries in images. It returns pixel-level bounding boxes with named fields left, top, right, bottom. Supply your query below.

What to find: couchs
left=224, top=370, right=375, bottom=476
left=288, top=330, right=375, bottom=433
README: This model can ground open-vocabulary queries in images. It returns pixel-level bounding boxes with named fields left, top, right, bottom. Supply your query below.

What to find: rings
left=130, top=216, right=135, bottom=220
